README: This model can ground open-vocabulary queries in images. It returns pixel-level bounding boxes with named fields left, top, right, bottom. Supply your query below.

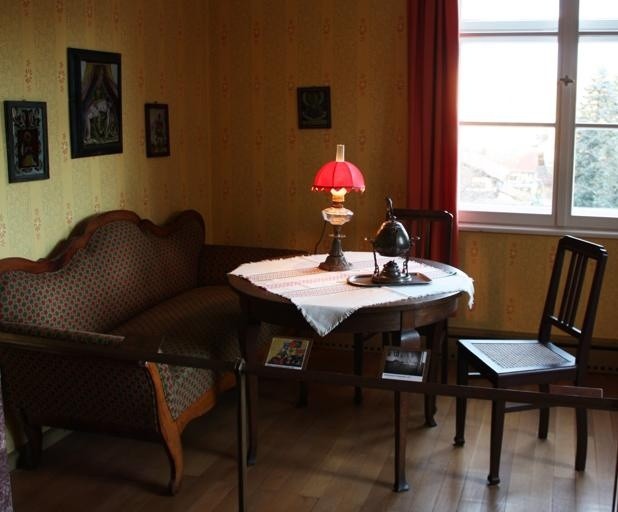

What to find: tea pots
left=374, top=197, right=412, bottom=256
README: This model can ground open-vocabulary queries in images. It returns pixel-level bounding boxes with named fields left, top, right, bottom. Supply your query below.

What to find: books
left=381, top=349, right=428, bottom=383
left=264, top=337, right=309, bottom=370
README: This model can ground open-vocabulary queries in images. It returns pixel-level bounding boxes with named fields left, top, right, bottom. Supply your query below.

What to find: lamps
left=310, top=143, right=366, bottom=273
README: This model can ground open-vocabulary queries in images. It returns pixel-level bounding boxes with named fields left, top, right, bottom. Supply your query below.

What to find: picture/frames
left=4, top=99, right=49, bottom=182
left=66, top=47, right=123, bottom=159
left=144, top=103, right=170, bottom=157
left=297, top=86, right=331, bottom=129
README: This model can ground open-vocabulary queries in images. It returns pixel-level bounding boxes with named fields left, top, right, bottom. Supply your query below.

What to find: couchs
left=1, top=209, right=278, bottom=496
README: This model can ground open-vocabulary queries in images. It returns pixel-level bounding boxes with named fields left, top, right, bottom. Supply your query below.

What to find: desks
left=227, top=251, right=474, bottom=494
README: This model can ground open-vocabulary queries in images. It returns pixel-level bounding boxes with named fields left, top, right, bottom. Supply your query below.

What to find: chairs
left=351, top=208, right=454, bottom=426
left=454, top=233, right=607, bottom=485
